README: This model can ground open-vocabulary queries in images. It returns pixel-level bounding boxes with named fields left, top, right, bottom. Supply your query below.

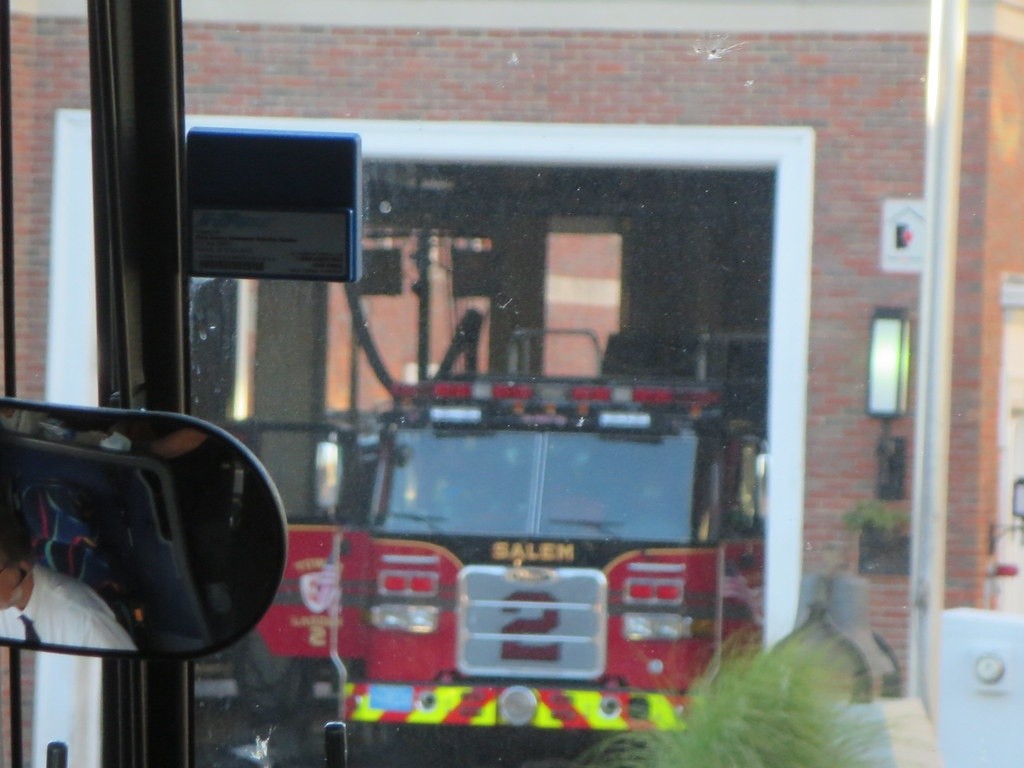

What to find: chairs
left=21, top=479, right=134, bottom=635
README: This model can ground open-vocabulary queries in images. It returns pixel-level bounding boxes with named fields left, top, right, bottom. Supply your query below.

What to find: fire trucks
left=222, top=328, right=768, bottom=733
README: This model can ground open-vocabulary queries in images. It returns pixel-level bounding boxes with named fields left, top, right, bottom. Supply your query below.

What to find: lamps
left=863, top=303, right=912, bottom=420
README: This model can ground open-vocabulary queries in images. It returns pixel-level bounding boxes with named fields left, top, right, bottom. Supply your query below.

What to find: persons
left=1, top=464, right=139, bottom=649
left=141, top=412, right=232, bottom=635
